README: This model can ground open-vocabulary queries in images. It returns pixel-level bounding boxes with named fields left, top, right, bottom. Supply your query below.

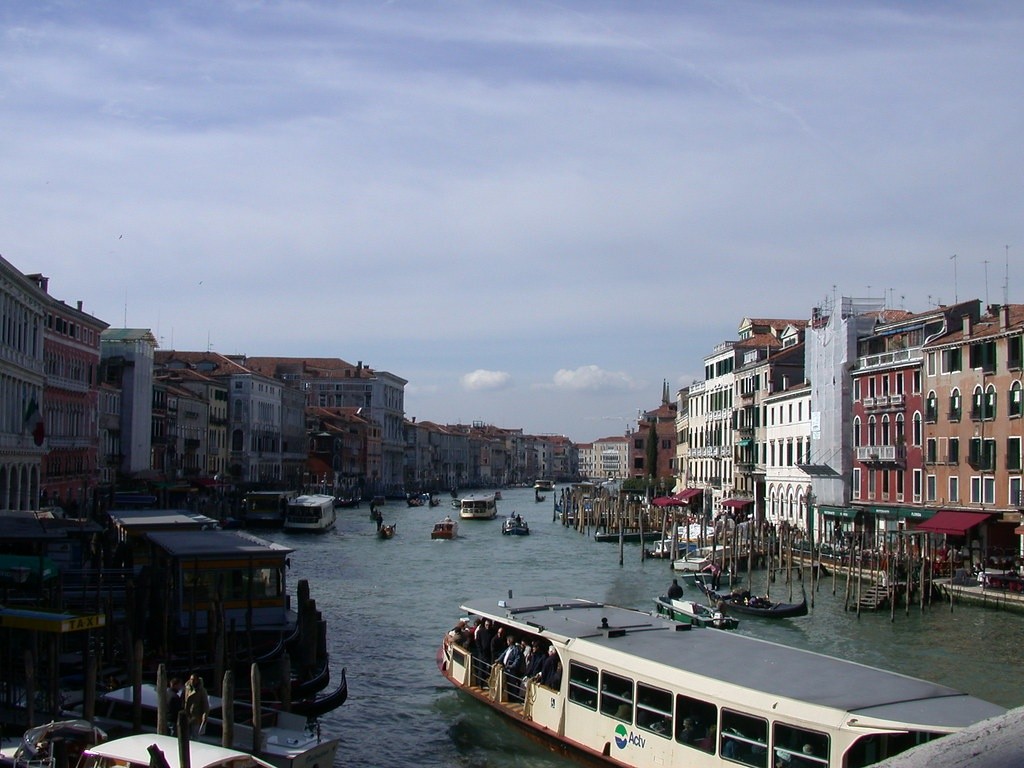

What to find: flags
left=21, top=397, right=45, bottom=447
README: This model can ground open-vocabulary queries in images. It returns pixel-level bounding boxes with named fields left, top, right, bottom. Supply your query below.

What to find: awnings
left=721, top=499, right=753, bottom=509
left=818, top=506, right=938, bottom=521
left=315, top=431, right=333, bottom=439
left=1014, top=525, right=1024, bottom=535
left=737, top=438, right=753, bottom=447
left=673, top=488, right=703, bottom=500
left=914, top=511, right=992, bottom=536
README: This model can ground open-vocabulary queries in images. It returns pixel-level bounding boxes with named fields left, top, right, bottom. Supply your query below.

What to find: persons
left=700, top=561, right=721, bottom=591
left=166, top=673, right=209, bottom=741
left=761, top=519, right=806, bottom=545
left=511, top=510, right=521, bottom=527
left=873, top=544, right=931, bottom=590
left=445, top=617, right=562, bottom=706
left=729, top=585, right=772, bottom=609
left=571, top=672, right=822, bottom=768
left=935, top=540, right=1024, bottom=595
left=879, top=554, right=888, bottom=591
left=710, top=594, right=727, bottom=618
left=369, top=500, right=375, bottom=513
left=668, top=579, right=683, bottom=605
left=445, top=515, right=452, bottom=522
left=716, top=510, right=754, bottom=526
left=375, top=513, right=383, bottom=531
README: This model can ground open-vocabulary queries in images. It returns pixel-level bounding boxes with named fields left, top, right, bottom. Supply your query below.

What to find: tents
left=653, top=496, right=688, bottom=508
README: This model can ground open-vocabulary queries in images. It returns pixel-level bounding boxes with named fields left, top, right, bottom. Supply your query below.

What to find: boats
left=673, top=545, right=730, bottom=572
left=0, top=507, right=350, bottom=768
left=533, top=480, right=555, bottom=491
left=459, top=494, right=497, bottom=520
left=245, top=491, right=300, bottom=526
left=501, top=517, right=529, bottom=535
left=430, top=520, right=459, bottom=539
left=369, top=489, right=461, bottom=539
left=491, top=491, right=502, bottom=500
left=650, top=595, right=740, bottom=631
left=433, top=589, right=1013, bottom=768
left=693, top=571, right=809, bottom=619
left=645, top=540, right=687, bottom=559
left=283, top=493, right=338, bottom=533
